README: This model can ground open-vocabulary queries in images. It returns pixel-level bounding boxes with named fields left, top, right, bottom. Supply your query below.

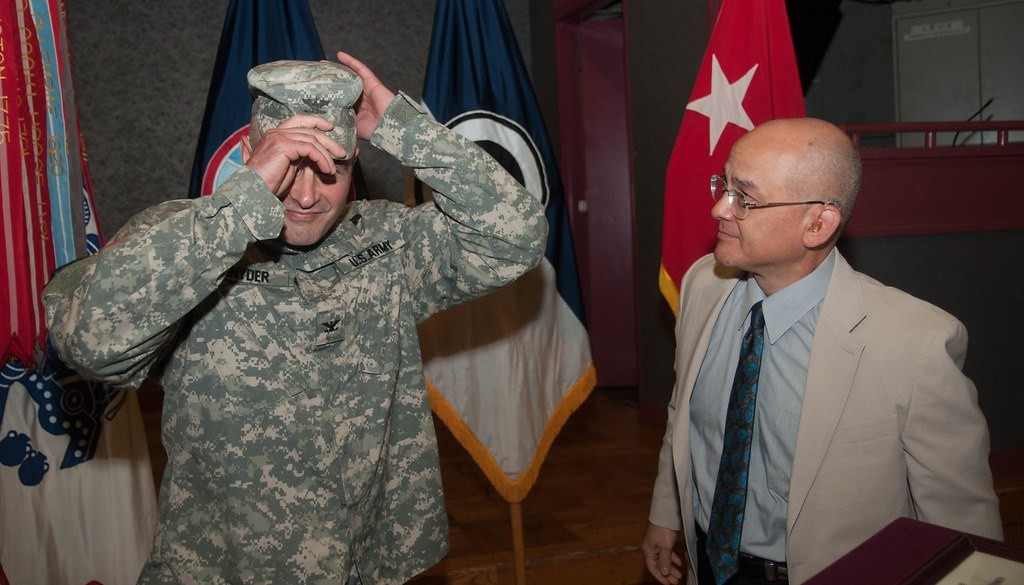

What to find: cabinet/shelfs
left=889, top=0, right=1024, bottom=149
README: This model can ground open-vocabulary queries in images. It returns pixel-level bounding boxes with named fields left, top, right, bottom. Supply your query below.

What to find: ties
left=705, top=299, right=765, bottom=585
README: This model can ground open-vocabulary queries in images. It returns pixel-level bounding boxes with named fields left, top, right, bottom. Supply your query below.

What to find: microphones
left=953, top=97, right=995, bottom=145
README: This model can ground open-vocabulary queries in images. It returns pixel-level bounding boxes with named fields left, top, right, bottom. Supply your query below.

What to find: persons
left=641, top=115, right=1005, bottom=585
left=41, top=51, right=550, bottom=585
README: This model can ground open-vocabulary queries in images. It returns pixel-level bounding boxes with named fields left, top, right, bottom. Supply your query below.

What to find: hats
left=247, top=60, right=364, bottom=161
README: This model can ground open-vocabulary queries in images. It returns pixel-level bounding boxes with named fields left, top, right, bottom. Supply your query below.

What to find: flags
left=405, top=0, right=597, bottom=506
left=187, top=0, right=373, bottom=202
left=0, top=0, right=158, bottom=585
left=657, top=1, right=808, bottom=319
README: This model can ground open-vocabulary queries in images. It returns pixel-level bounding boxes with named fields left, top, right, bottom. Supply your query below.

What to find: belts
left=694, top=519, right=790, bottom=585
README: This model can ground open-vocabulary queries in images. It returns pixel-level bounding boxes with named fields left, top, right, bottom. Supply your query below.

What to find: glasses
left=710, top=175, right=826, bottom=219
